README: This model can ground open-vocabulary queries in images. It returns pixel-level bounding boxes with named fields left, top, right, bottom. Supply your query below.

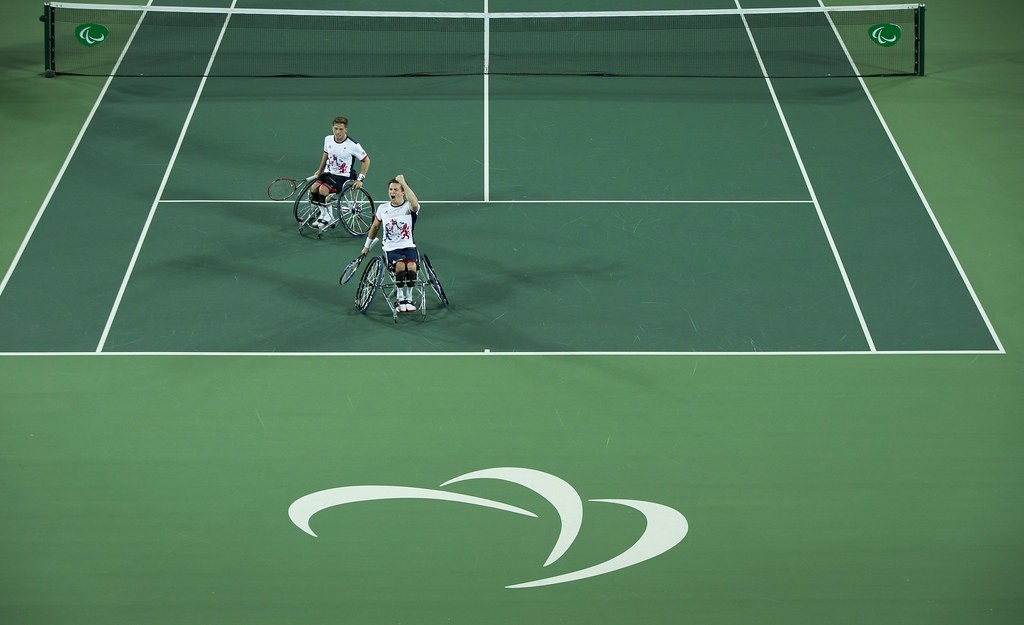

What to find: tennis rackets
left=268, top=175, right=316, bottom=201
left=340, top=238, right=380, bottom=286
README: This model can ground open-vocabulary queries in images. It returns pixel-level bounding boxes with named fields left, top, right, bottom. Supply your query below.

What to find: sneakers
left=397, top=301, right=416, bottom=312
left=311, top=214, right=333, bottom=228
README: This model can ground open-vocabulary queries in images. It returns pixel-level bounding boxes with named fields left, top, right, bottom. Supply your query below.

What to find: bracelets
left=357, top=173, right=365, bottom=182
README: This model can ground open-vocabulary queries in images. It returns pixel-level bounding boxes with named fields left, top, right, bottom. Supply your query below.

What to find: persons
left=310, top=117, right=370, bottom=227
left=361, top=175, right=420, bottom=312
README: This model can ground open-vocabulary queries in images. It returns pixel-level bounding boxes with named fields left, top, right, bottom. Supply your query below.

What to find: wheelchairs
left=293, top=169, right=375, bottom=240
left=355, top=248, right=449, bottom=323
